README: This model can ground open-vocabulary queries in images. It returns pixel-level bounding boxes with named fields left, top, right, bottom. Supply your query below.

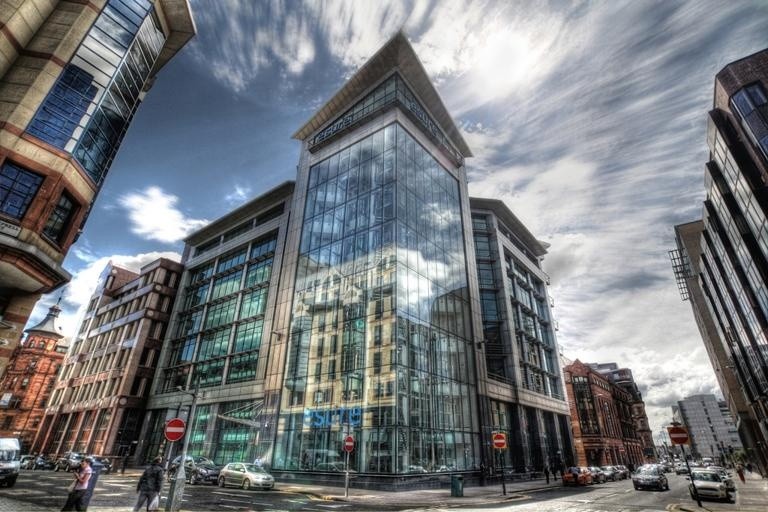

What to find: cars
left=0, top=434, right=113, bottom=489
left=631, top=453, right=734, bottom=497
left=168, top=455, right=276, bottom=492
left=557, top=462, right=631, bottom=485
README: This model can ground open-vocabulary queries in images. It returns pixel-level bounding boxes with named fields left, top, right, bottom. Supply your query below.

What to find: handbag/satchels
left=67, top=479, right=79, bottom=492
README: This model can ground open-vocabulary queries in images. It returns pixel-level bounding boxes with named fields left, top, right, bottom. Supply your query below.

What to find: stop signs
left=667, top=423, right=690, bottom=449
left=164, top=419, right=186, bottom=442
left=491, top=432, right=507, bottom=449
left=343, top=436, right=355, bottom=453
